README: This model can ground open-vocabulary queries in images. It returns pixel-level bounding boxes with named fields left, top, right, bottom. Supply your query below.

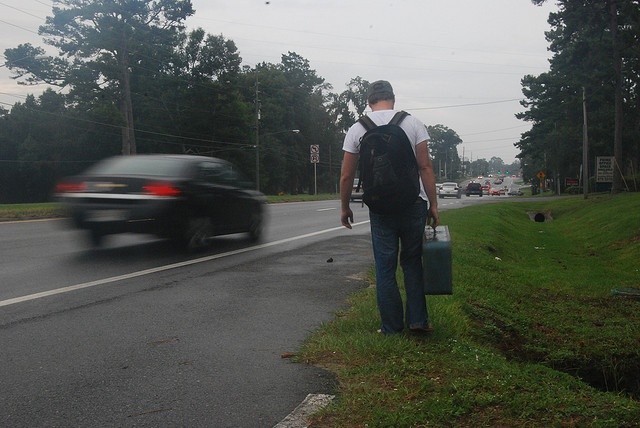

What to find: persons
left=341, top=80, right=440, bottom=334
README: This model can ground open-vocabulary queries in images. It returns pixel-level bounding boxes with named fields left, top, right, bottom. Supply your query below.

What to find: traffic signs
left=310, top=153, right=320, bottom=164
left=309, top=144, right=319, bottom=153
left=536, top=171, right=545, bottom=180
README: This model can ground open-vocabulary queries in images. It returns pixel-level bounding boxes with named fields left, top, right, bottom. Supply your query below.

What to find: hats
left=366, top=80, right=393, bottom=98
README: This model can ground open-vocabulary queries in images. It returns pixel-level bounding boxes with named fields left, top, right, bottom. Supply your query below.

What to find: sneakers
left=409, top=322, right=432, bottom=332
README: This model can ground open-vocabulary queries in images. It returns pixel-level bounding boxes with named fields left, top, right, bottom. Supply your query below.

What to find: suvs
left=464, top=183, right=483, bottom=198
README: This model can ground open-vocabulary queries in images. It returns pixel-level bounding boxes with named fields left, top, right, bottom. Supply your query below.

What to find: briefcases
left=423, top=225, right=452, bottom=294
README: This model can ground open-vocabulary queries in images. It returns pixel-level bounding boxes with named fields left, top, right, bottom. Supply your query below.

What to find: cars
left=483, top=174, right=523, bottom=197
left=350, top=178, right=364, bottom=202
left=53, top=153, right=266, bottom=256
left=477, top=175, right=482, bottom=179
left=439, top=183, right=462, bottom=199
